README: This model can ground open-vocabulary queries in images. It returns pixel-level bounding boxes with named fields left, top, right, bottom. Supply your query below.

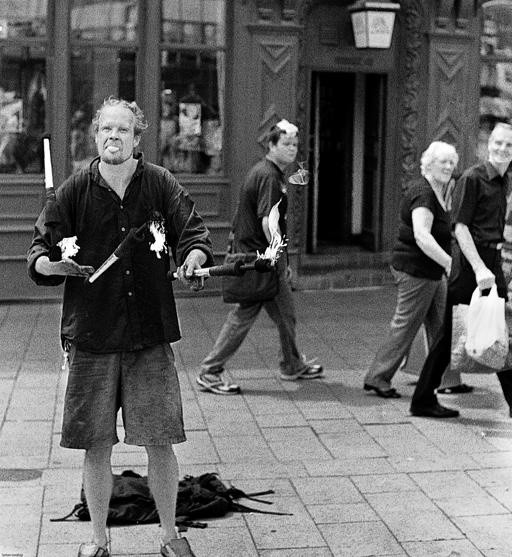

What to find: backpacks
left=77, top=470, right=253, bottom=532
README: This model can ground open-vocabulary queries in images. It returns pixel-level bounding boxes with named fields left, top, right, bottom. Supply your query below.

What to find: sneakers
left=280, top=364, right=322, bottom=380
left=197, top=373, right=241, bottom=395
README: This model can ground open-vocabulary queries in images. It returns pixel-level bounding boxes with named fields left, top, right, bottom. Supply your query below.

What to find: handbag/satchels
left=222, top=253, right=280, bottom=303
left=465, top=282, right=510, bottom=369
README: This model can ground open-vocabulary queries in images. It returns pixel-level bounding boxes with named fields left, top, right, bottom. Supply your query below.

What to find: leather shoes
left=366, top=384, right=472, bottom=418
left=78, top=543, right=109, bottom=557
left=161, top=537, right=195, bottom=557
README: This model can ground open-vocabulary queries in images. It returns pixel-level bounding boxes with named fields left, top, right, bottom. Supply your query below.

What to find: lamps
left=348, top=0, right=401, bottom=50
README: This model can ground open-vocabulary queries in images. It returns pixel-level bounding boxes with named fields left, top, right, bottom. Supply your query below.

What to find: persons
left=361, top=138, right=475, bottom=401
left=193, top=116, right=328, bottom=398
left=1, top=76, right=223, bottom=178
left=406, top=119, right=512, bottom=420
left=27, top=97, right=215, bottom=556
